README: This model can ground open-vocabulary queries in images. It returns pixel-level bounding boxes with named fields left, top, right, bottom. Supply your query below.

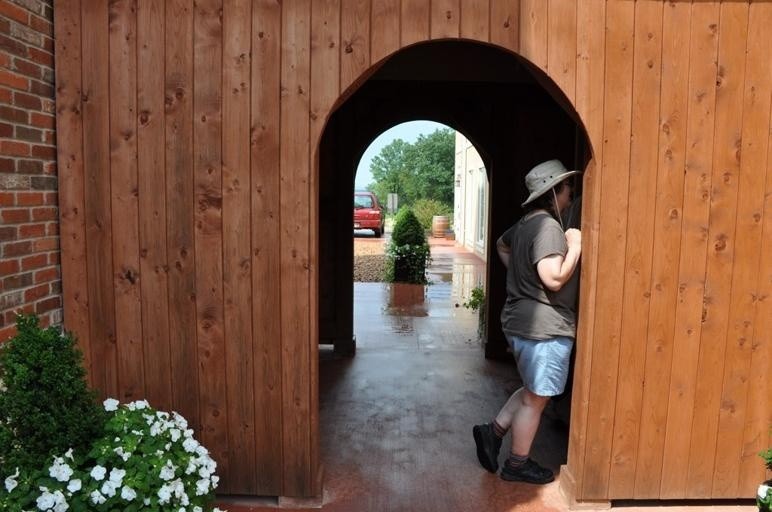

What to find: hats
left=522, top=160, right=583, bottom=205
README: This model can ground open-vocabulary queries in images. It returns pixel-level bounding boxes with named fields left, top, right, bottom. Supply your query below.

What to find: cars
left=353, top=192, right=386, bottom=237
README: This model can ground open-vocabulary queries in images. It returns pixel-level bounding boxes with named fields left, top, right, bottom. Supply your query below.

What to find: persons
left=473, top=159, right=582, bottom=484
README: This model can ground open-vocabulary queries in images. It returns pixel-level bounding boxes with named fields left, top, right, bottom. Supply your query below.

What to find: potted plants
left=756, top=449, right=772, bottom=512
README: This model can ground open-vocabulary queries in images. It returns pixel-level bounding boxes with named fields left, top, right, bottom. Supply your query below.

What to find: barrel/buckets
left=431, top=216, right=449, bottom=238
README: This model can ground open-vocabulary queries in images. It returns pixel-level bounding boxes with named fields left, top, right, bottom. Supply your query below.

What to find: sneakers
left=474, top=423, right=502, bottom=473
left=501, top=459, right=554, bottom=482
